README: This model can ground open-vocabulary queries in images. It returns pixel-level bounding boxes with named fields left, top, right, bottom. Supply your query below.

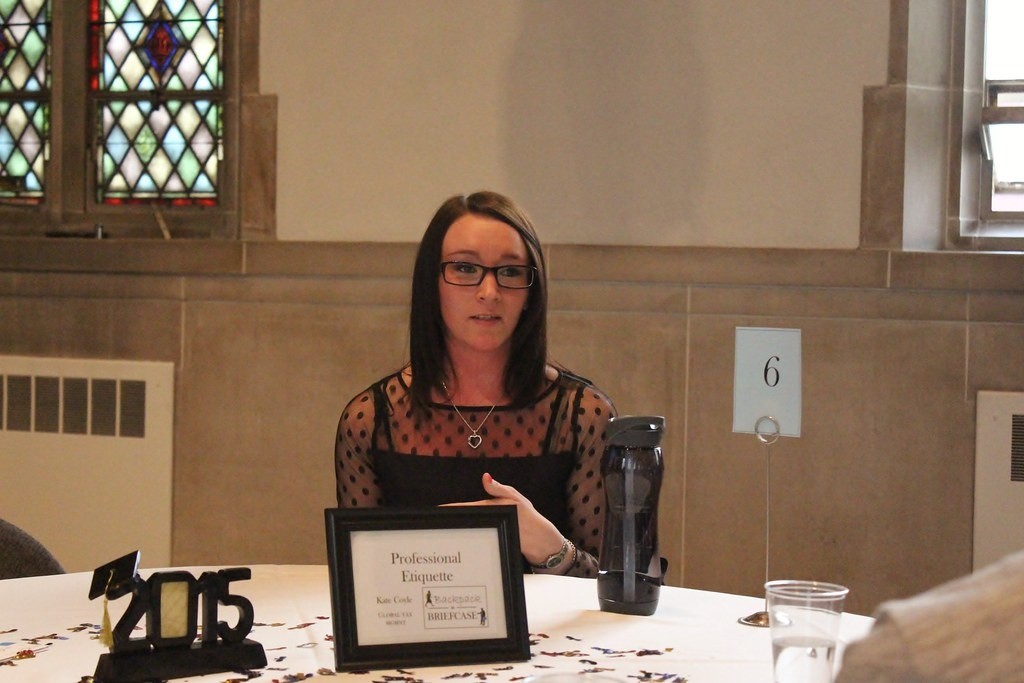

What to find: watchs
left=531, top=537, right=569, bottom=569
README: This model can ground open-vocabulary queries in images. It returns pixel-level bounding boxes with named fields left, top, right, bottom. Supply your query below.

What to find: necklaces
left=442, top=380, right=505, bottom=449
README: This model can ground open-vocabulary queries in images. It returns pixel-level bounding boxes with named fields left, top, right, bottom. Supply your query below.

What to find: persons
left=333, top=191, right=618, bottom=581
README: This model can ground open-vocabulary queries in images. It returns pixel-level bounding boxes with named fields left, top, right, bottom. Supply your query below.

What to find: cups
left=764, top=577, right=848, bottom=682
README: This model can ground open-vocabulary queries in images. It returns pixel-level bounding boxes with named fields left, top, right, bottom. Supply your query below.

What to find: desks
left=0, top=565, right=878, bottom=683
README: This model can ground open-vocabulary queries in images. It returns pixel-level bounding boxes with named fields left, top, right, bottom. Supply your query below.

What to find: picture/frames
left=326, top=504, right=533, bottom=671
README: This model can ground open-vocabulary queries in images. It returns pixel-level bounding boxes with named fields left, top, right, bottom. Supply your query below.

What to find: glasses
left=435, top=260, right=539, bottom=290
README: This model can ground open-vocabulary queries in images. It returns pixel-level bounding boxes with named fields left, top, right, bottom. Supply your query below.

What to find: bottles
left=598, top=415, right=665, bottom=616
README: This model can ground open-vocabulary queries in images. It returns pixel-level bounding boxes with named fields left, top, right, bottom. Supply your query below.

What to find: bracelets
left=532, top=539, right=576, bottom=575
